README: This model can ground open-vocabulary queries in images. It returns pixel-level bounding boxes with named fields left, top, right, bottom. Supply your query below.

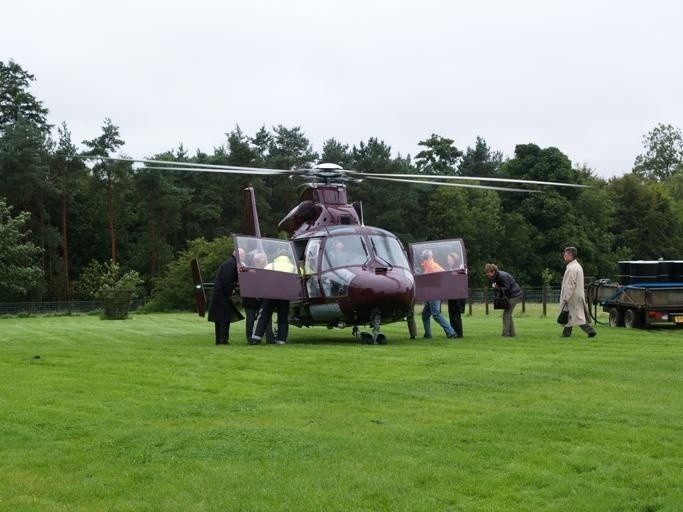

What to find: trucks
left=592, top=261, right=683, bottom=328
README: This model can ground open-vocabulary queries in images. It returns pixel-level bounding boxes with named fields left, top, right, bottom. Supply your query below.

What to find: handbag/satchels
left=494, top=297, right=511, bottom=309
left=557, top=311, right=569, bottom=324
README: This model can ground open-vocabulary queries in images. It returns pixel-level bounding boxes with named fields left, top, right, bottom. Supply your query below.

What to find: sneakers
left=588, top=332, right=597, bottom=337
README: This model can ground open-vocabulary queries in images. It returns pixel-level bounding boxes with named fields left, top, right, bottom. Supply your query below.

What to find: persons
left=208, top=248, right=245, bottom=344
left=329, top=242, right=344, bottom=263
left=407, top=264, right=420, bottom=339
left=444, top=252, right=467, bottom=337
left=558, top=247, right=597, bottom=338
left=241, top=253, right=276, bottom=344
left=484, top=263, right=523, bottom=337
left=252, top=249, right=303, bottom=345
left=418, top=248, right=458, bottom=339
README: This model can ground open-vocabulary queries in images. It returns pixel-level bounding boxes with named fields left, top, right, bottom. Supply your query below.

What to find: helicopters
left=52, top=153, right=597, bottom=346
